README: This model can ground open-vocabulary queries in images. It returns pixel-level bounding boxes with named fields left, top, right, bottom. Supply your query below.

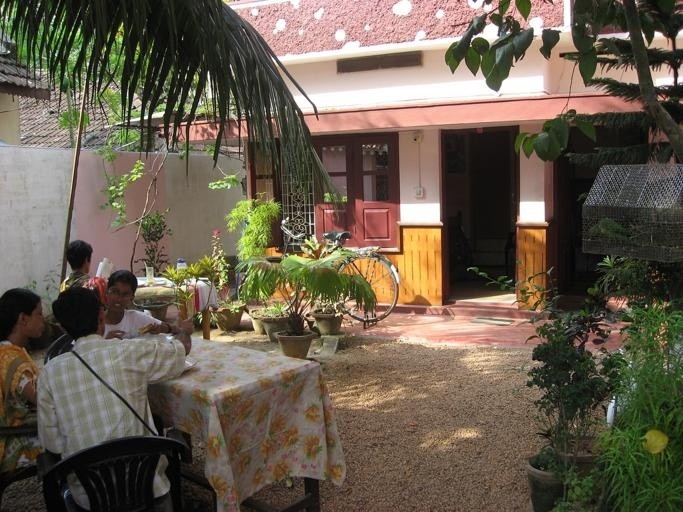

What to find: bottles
left=606, top=396, right=618, bottom=428
left=177, top=258, right=189, bottom=275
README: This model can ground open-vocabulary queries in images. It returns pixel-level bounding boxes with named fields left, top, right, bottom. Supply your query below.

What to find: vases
left=207, top=302, right=245, bottom=332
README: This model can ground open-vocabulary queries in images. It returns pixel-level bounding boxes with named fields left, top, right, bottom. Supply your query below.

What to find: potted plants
left=224, top=192, right=377, bottom=359
left=464, top=264, right=623, bottom=512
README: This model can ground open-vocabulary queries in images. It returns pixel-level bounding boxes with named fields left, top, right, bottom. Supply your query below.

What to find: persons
left=100, top=269, right=180, bottom=341
left=35, top=285, right=194, bottom=510
left=0, top=287, right=47, bottom=476
left=57, top=239, right=107, bottom=316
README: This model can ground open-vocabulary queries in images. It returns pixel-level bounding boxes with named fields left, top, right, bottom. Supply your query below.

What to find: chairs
left=44, top=334, right=74, bottom=363
left=0, top=421, right=192, bottom=512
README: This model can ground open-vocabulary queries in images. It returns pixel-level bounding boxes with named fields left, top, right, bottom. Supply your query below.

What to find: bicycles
left=231, top=216, right=403, bottom=334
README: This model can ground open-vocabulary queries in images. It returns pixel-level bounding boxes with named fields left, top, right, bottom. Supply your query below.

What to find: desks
left=148, top=337, right=321, bottom=511
left=134, top=276, right=212, bottom=340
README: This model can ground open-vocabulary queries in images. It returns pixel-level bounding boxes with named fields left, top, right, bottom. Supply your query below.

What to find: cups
left=145, top=266, right=155, bottom=286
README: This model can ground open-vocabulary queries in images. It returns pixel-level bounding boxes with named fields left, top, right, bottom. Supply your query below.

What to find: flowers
left=209, top=229, right=232, bottom=306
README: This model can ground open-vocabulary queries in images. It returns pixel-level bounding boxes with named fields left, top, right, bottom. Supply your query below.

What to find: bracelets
left=166, top=323, right=171, bottom=333
